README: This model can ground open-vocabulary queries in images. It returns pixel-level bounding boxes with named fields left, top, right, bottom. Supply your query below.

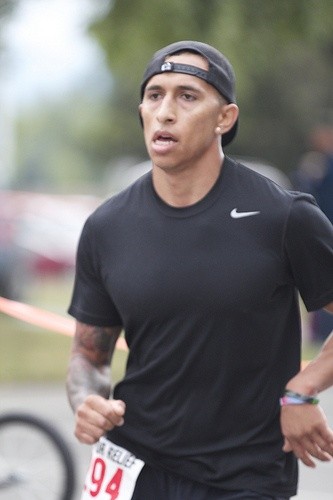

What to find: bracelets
left=283, top=389, right=319, bottom=405
left=277, top=396, right=309, bottom=406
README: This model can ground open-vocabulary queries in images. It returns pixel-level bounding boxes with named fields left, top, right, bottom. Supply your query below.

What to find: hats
left=140, top=41, right=238, bottom=145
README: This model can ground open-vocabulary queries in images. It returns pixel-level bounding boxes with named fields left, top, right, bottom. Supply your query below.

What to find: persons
left=62, top=39, right=333, bottom=500
